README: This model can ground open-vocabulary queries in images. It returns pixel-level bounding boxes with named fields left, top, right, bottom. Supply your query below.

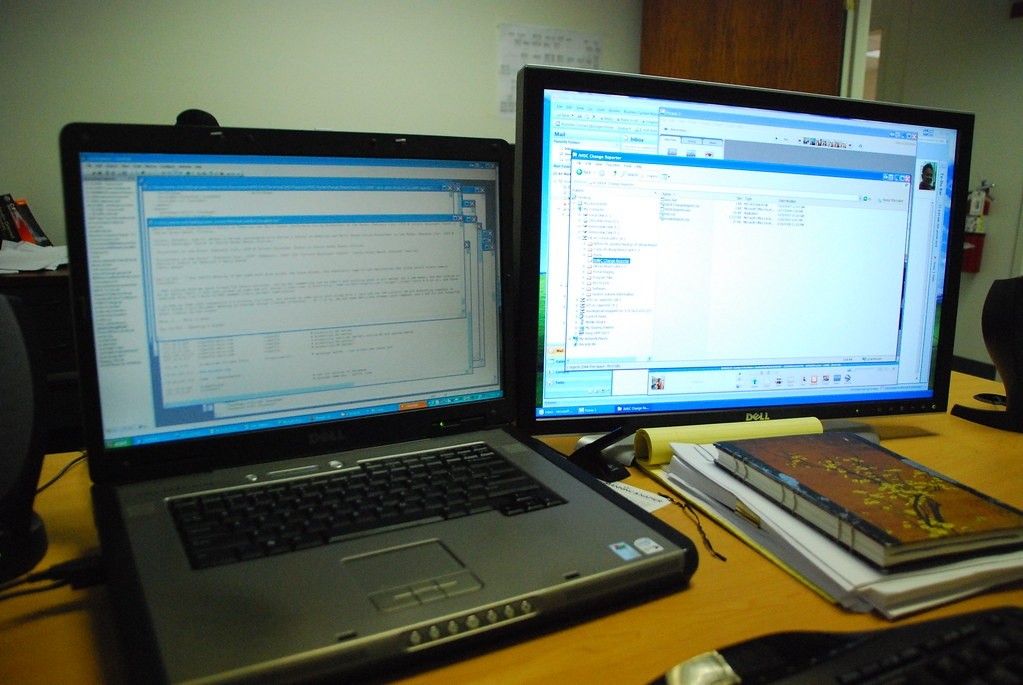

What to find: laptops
left=53, top=117, right=698, bottom=685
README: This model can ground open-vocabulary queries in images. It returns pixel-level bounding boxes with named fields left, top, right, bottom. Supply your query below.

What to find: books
left=0, top=193, right=55, bottom=249
left=634, top=431, right=1023, bottom=620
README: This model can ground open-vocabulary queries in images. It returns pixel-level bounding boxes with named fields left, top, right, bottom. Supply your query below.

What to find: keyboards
left=774, top=607, right=1023, bottom=685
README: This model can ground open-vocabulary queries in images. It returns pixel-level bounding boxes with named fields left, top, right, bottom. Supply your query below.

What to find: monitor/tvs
left=512, top=67, right=973, bottom=483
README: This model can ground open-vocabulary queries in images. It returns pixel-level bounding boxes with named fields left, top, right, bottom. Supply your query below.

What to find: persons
left=919, top=164, right=934, bottom=190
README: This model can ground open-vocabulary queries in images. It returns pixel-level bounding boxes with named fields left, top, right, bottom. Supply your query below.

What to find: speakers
left=0, top=294, right=48, bottom=584
left=951, top=276, right=1022, bottom=434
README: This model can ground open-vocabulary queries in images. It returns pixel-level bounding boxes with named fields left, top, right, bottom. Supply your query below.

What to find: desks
left=0, top=371, right=1023, bottom=684
